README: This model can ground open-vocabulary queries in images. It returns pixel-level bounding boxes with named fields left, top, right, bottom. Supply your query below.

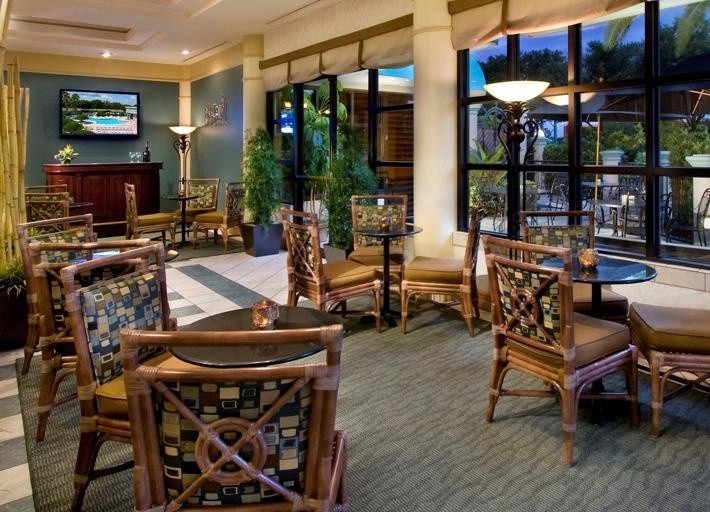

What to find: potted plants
left=239, top=127, right=284, bottom=257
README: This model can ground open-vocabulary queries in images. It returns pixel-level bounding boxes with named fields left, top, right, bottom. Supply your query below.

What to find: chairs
left=401, top=206, right=481, bottom=337
left=481, top=235, right=639, bottom=465
left=120, top=325, right=348, bottom=512
left=519, top=188, right=710, bottom=323
left=629, top=303, right=710, bottom=435
left=281, top=207, right=381, bottom=331
left=348, top=194, right=408, bottom=303
left=61, top=241, right=177, bottom=511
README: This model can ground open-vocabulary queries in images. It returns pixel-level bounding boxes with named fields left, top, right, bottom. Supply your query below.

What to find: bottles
left=143, top=140, right=151, bottom=162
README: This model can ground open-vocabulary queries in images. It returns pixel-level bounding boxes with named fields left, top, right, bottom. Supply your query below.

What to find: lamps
left=484, top=80, right=551, bottom=241
left=169, top=126, right=198, bottom=197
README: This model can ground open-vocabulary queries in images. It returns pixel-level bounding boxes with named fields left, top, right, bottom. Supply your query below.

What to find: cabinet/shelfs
left=42, top=162, right=164, bottom=238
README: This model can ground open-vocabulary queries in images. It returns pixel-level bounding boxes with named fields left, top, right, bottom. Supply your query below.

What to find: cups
left=598, top=179, right=601, bottom=185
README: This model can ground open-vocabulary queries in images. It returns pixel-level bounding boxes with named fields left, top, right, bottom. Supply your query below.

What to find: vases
left=65, top=159, right=71, bottom=164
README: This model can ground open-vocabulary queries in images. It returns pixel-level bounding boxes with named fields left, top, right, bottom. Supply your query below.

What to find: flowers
left=54, top=145, right=78, bottom=162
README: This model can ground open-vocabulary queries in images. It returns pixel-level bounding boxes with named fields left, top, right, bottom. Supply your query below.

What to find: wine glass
left=128, top=152, right=135, bottom=163
left=136, top=152, right=141, bottom=163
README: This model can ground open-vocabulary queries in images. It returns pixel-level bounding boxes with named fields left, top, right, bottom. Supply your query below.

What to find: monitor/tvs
left=280, top=109, right=294, bottom=134
left=60, top=88, right=140, bottom=138
